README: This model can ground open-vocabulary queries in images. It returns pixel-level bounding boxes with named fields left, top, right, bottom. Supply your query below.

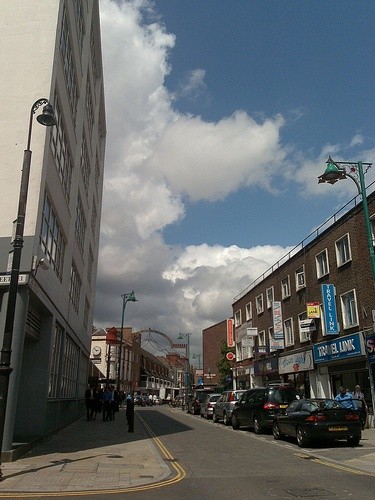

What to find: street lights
left=0, top=98, right=58, bottom=460
left=317, top=155, right=375, bottom=296
left=116, top=288, right=139, bottom=394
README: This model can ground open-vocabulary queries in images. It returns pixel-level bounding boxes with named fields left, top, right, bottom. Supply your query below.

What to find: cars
left=133, top=387, right=214, bottom=415
left=272, top=398, right=367, bottom=448
left=199, top=394, right=223, bottom=419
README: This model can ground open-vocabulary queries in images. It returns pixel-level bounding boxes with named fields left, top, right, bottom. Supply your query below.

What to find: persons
left=352, top=385, right=369, bottom=430
left=85, top=384, right=127, bottom=422
left=335, top=385, right=358, bottom=410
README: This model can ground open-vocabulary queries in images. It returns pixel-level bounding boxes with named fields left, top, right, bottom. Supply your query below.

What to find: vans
left=231, top=386, right=302, bottom=434
left=213, top=389, right=250, bottom=426
